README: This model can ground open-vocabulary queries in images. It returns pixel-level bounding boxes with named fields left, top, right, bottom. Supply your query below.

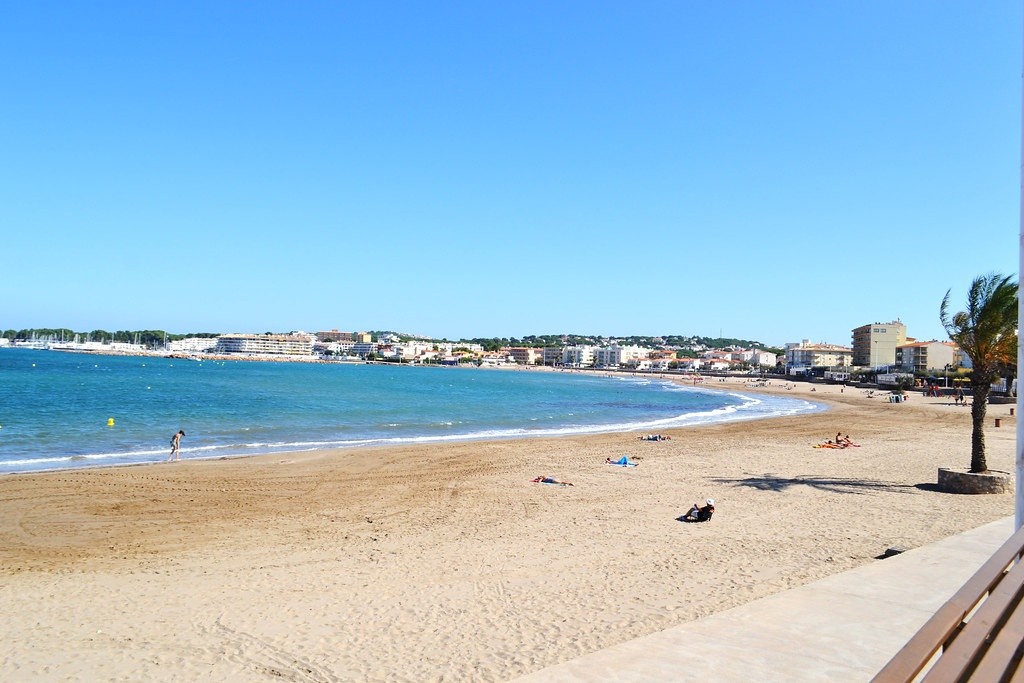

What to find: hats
left=707, top=498, right=714, bottom=506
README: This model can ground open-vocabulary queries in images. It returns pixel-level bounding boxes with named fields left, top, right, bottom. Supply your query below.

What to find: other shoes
left=680, top=515, right=687, bottom=520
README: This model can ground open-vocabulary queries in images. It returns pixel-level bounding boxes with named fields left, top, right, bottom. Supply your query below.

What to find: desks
left=890, top=395, right=906, bottom=403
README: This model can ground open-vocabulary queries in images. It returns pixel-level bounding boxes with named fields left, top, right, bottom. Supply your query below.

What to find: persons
left=605, top=455, right=638, bottom=466
left=694, top=377, right=968, bottom=405
left=538, top=475, right=575, bottom=486
left=168, top=430, right=185, bottom=461
left=818, top=431, right=861, bottom=449
left=679, top=499, right=714, bottom=521
left=637, top=434, right=671, bottom=441
